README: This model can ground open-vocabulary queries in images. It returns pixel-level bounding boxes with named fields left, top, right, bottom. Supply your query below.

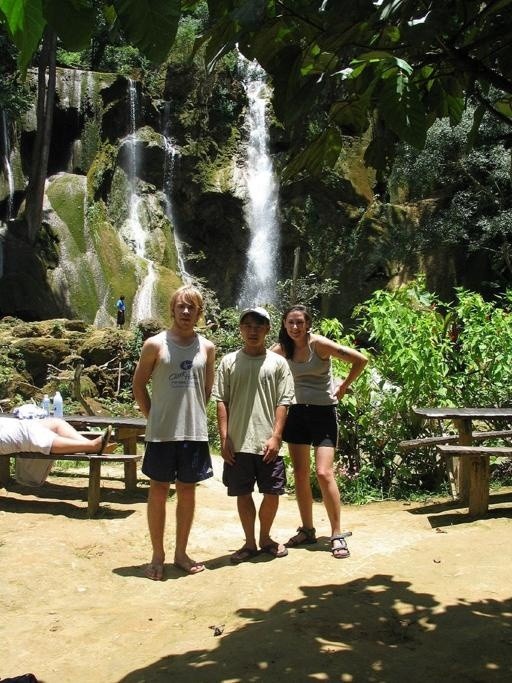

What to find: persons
left=132, top=285, right=216, bottom=581
left=213, top=306, right=297, bottom=563
left=0, top=417, right=118, bottom=456
left=271, top=305, right=368, bottom=558
left=116, top=295, right=125, bottom=330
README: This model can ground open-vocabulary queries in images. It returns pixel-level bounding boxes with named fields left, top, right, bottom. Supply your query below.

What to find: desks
left=63, top=416, right=147, bottom=494
left=411, top=405, right=512, bottom=504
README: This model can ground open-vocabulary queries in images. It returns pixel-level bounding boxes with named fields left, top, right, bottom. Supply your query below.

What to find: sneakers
left=97, top=424, right=113, bottom=456
left=103, top=442, right=118, bottom=454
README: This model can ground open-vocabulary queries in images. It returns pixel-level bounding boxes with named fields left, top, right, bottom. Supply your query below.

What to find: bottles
left=42, top=394, right=51, bottom=417
left=53, top=391, right=63, bottom=417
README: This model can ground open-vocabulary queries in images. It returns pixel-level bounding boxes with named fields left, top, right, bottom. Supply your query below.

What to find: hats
left=239, top=305, right=271, bottom=324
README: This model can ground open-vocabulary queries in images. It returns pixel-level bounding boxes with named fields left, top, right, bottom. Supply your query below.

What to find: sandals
left=230, top=544, right=258, bottom=565
left=330, top=535, right=351, bottom=560
left=260, top=541, right=288, bottom=558
left=174, top=557, right=206, bottom=574
left=286, top=526, right=317, bottom=547
left=144, top=553, right=165, bottom=581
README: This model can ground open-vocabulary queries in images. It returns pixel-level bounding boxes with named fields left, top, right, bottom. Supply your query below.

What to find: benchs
left=401, top=430, right=512, bottom=517
left=1, top=431, right=145, bottom=516
